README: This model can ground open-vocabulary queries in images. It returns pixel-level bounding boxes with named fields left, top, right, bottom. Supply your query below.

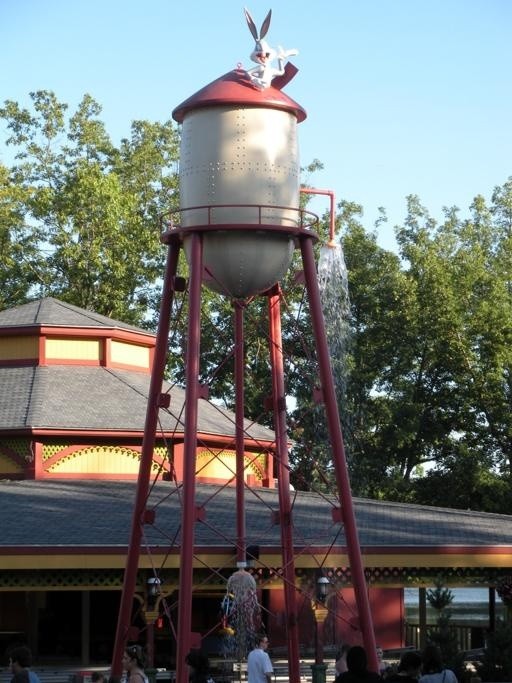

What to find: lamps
left=146, top=577, right=161, bottom=596
left=317, top=577, right=329, bottom=601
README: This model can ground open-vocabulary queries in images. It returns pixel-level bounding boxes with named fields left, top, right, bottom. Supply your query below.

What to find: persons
left=5, top=643, right=44, bottom=683
left=120, top=644, right=150, bottom=683
left=333, top=642, right=458, bottom=682
left=89, top=671, right=106, bottom=683
left=245, top=635, right=275, bottom=683
left=184, top=648, right=216, bottom=683
